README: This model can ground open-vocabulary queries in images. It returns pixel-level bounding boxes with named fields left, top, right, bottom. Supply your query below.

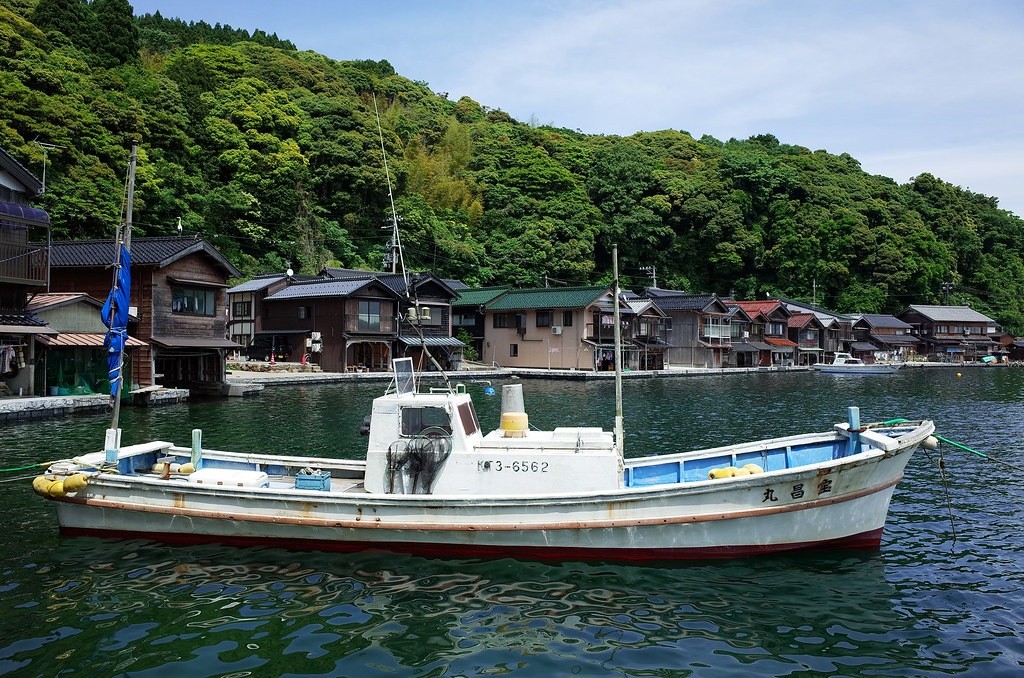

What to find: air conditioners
left=912, top=330, right=918, bottom=334
left=921, top=330, right=927, bottom=334
left=552, top=326, right=562, bottom=334
left=742, top=331, right=749, bottom=338
left=965, top=330, right=970, bottom=335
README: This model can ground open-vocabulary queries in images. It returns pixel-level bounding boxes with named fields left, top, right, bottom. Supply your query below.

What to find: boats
left=31, top=87, right=937, bottom=567
left=812, top=352, right=904, bottom=374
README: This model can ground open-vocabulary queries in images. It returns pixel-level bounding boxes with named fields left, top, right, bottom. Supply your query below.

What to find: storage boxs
left=295, top=471, right=331, bottom=492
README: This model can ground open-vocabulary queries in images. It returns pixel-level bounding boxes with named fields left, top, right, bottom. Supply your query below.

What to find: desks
left=347, top=366, right=366, bottom=373
